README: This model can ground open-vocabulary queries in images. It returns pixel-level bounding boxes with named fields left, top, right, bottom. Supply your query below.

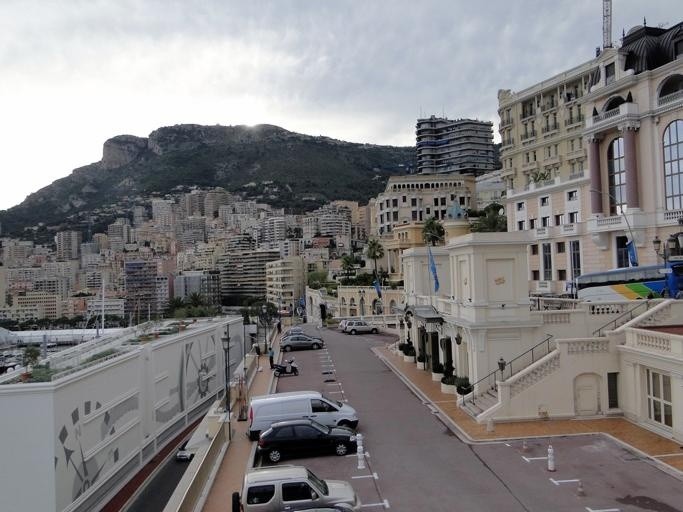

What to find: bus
left=566, top=261, right=683, bottom=301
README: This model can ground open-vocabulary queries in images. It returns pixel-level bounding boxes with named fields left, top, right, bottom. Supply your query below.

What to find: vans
left=245, top=391, right=359, bottom=441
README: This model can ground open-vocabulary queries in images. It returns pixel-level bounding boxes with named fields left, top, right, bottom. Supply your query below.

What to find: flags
left=428, top=249, right=440, bottom=292
left=373, top=271, right=382, bottom=298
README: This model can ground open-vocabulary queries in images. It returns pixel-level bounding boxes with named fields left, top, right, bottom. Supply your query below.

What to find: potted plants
left=397, top=343, right=471, bottom=401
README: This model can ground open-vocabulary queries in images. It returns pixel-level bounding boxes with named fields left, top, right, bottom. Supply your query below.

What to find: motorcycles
left=273, top=357, right=298, bottom=376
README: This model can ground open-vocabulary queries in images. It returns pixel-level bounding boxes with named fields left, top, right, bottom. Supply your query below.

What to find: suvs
left=232, top=465, right=361, bottom=512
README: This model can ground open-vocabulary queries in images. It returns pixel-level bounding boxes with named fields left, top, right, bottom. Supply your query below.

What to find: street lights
left=652, top=235, right=676, bottom=298
left=261, top=303, right=269, bottom=354
left=221, top=330, right=231, bottom=412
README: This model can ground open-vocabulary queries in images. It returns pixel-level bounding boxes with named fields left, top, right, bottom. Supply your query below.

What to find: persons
left=269, top=348, right=275, bottom=369
left=251, top=342, right=261, bottom=356
left=646, top=292, right=654, bottom=299
left=276, top=321, right=282, bottom=334
left=660, top=287, right=666, bottom=298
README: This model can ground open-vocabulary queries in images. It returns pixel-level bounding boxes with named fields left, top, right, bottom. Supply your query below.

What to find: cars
left=280, top=331, right=325, bottom=351
left=176, top=440, right=193, bottom=460
left=337, top=319, right=380, bottom=335
left=258, top=419, right=357, bottom=463
